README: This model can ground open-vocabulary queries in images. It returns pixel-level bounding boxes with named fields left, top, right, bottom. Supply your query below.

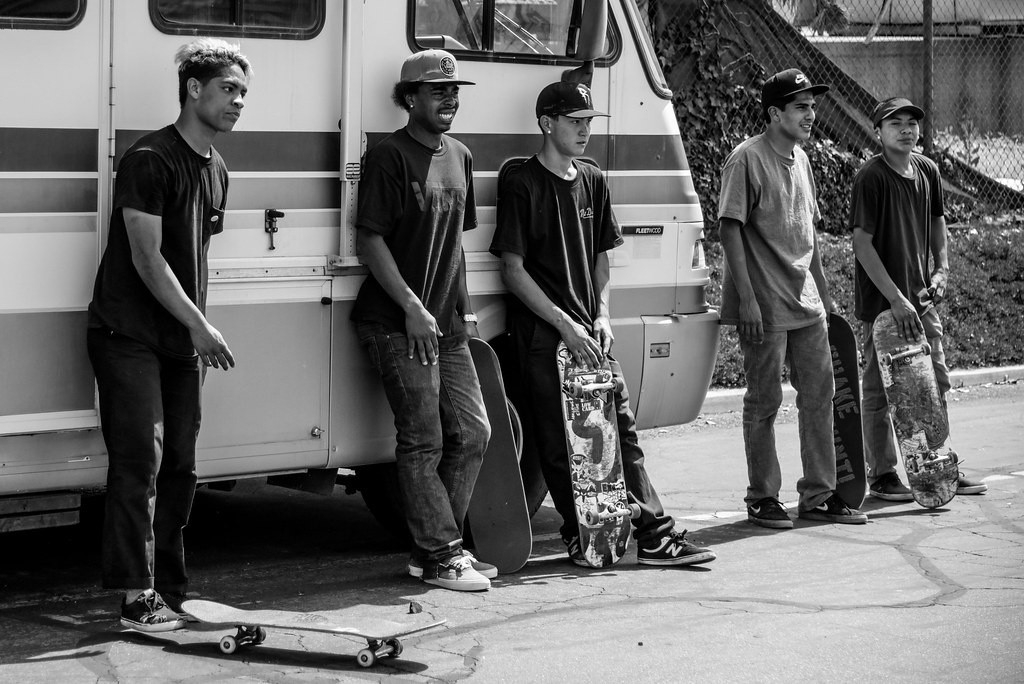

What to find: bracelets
left=460, top=312, right=477, bottom=324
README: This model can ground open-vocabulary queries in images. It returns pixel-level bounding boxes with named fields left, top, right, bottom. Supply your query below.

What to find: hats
left=761, top=65, right=831, bottom=102
left=399, top=47, right=475, bottom=84
left=869, top=97, right=928, bottom=122
left=536, top=82, right=614, bottom=120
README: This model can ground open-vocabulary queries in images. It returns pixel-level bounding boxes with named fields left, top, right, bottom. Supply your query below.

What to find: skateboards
left=871, top=307, right=960, bottom=509
left=825, top=311, right=871, bottom=512
left=179, top=599, right=449, bottom=669
left=556, top=334, right=644, bottom=568
left=450, top=337, right=533, bottom=576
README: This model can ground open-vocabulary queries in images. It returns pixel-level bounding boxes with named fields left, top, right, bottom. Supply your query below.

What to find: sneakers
left=165, top=592, right=204, bottom=624
left=420, top=556, right=493, bottom=593
left=868, top=469, right=917, bottom=503
left=746, top=497, right=797, bottom=529
left=563, top=534, right=594, bottom=567
left=121, top=588, right=186, bottom=633
left=956, top=460, right=991, bottom=494
left=803, top=492, right=870, bottom=524
left=635, top=527, right=718, bottom=568
left=408, top=548, right=501, bottom=582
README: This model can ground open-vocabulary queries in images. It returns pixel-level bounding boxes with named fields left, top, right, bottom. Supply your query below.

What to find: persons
left=351, top=55, right=498, bottom=592
left=488, top=79, right=718, bottom=565
left=718, top=67, right=869, bottom=529
left=87, top=38, right=253, bottom=631
left=846, top=97, right=988, bottom=500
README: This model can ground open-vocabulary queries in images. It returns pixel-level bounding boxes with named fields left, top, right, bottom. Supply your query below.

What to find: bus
left=1, top=0, right=720, bottom=548
